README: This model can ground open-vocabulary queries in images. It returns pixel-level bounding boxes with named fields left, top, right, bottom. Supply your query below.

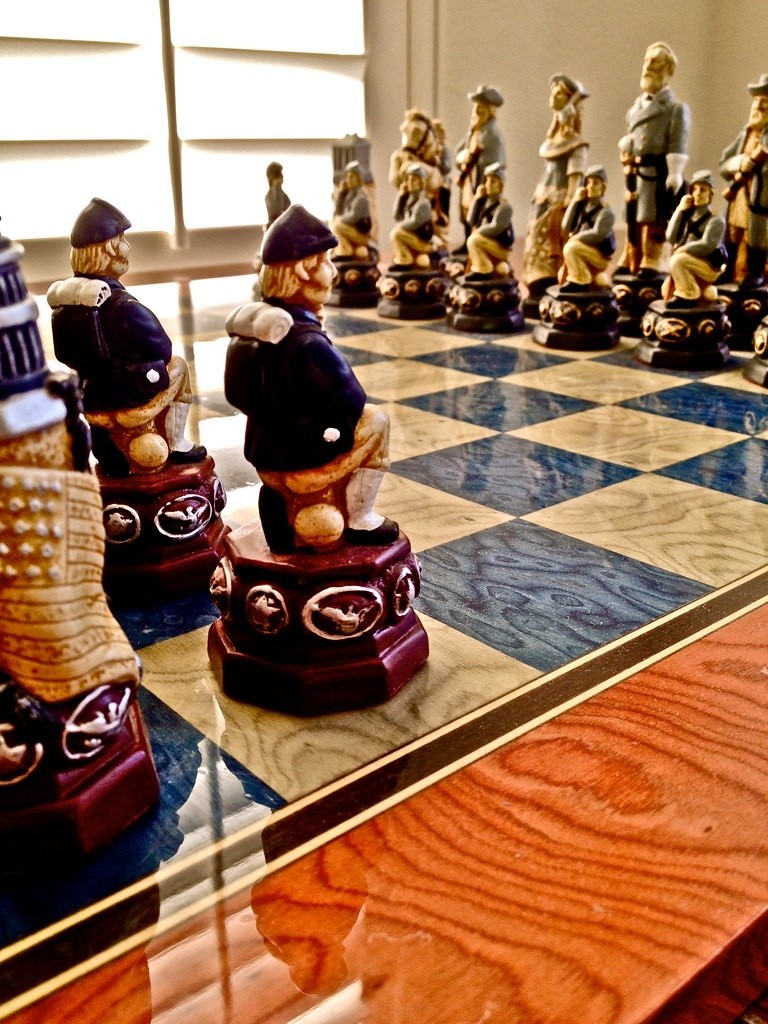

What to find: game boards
left=0, top=273, right=768, bottom=1024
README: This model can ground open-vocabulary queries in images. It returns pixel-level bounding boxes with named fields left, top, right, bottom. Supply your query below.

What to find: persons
left=561, top=165, right=614, bottom=292
left=224, top=204, right=401, bottom=555
left=329, top=161, right=381, bottom=261
left=455, top=86, right=515, bottom=282
left=389, top=106, right=453, bottom=271
left=524, top=71, right=590, bottom=278
left=663, top=169, right=731, bottom=310
left=613, top=42, right=691, bottom=280
left=714, top=74, right=767, bottom=289
left=266, top=163, right=291, bottom=225
left=46, top=198, right=207, bottom=479
